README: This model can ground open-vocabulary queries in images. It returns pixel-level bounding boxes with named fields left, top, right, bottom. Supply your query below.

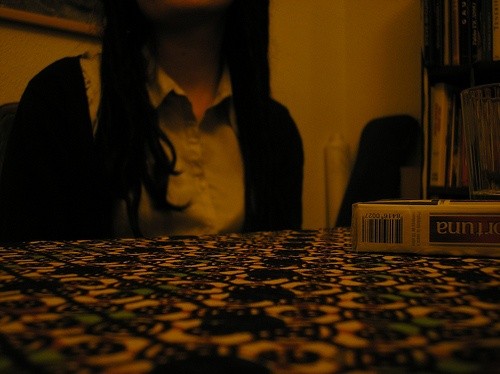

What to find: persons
left=0, top=0, right=307, bottom=247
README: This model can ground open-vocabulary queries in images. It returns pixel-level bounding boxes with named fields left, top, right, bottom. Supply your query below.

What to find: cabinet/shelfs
left=420, top=0, right=500, bottom=202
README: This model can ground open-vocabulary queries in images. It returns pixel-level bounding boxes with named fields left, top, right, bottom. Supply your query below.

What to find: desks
left=1, top=228, right=500, bottom=374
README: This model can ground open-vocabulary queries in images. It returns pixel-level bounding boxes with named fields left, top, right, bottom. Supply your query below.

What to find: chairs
left=1, top=104, right=16, bottom=172
